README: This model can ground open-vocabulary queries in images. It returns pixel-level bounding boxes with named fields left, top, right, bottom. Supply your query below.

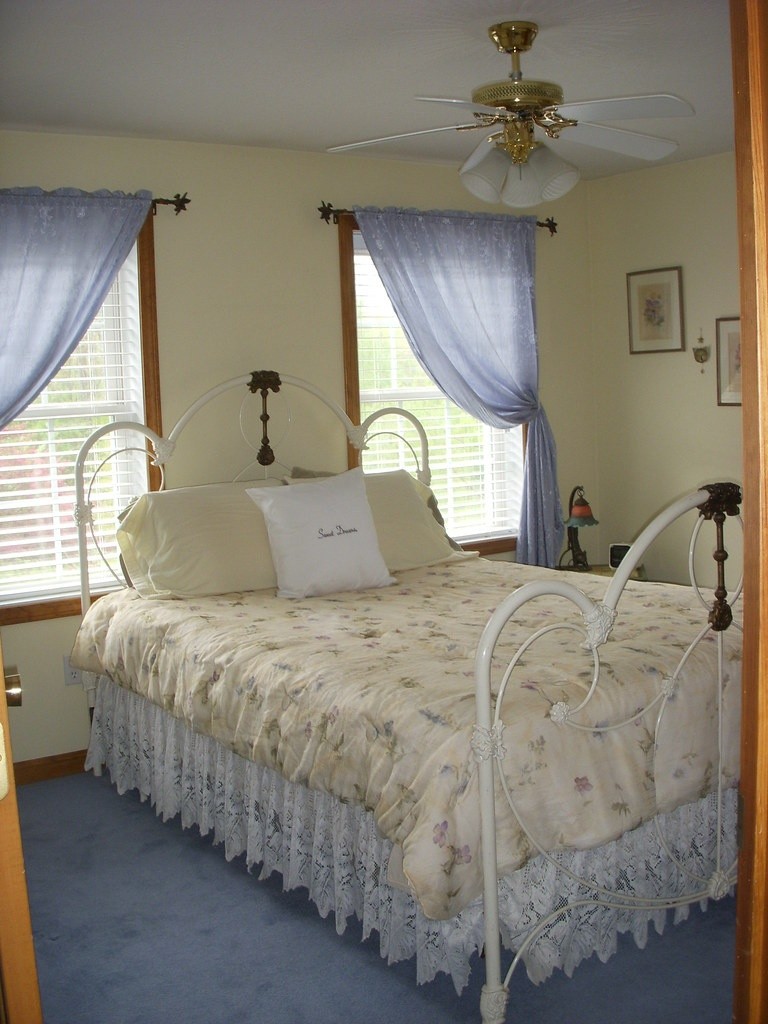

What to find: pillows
left=245, top=467, right=398, bottom=601
left=116, top=477, right=283, bottom=601
left=280, top=469, right=481, bottom=575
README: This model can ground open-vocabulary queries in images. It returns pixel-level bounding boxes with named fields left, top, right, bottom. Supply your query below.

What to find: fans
left=326, top=21, right=696, bottom=175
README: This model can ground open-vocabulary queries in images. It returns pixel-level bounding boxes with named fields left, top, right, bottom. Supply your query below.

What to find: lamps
left=458, top=111, right=581, bottom=207
left=558, top=483, right=599, bottom=569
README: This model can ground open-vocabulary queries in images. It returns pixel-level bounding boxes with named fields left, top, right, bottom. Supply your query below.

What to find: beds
left=76, top=371, right=745, bottom=1024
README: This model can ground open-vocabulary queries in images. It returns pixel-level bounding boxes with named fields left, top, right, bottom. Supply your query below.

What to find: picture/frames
left=626, top=266, right=686, bottom=355
left=715, top=317, right=743, bottom=406
